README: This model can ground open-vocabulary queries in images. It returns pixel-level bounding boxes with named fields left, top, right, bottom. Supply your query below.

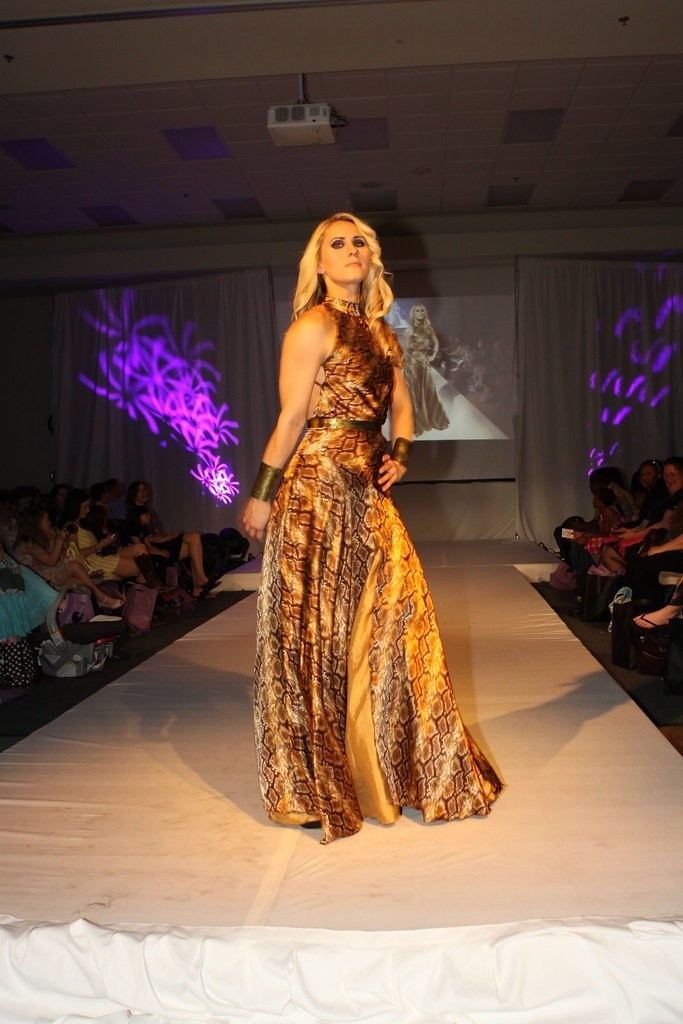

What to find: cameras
left=61, top=524, right=78, bottom=534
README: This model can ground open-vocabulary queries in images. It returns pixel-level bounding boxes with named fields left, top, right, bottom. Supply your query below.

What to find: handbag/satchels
left=636, top=629, right=671, bottom=676
left=122, top=585, right=158, bottom=635
left=35, top=640, right=113, bottom=678
left=0, top=639, right=35, bottom=694
left=550, top=561, right=578, bottom=592
left=611, top=602, right=652, bottom=671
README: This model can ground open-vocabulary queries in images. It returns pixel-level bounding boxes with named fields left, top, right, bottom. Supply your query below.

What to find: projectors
left=266, top=102, right=337, bottom=146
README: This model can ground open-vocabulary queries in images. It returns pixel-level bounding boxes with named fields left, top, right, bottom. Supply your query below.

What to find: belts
left=307, top=417, right=382, bottom=433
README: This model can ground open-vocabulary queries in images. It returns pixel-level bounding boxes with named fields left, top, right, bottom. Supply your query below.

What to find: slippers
left=198, top=577, right=222, bottom=592
left=191, top=589, right=215, bottom=601
left=630, top=611, right=672, bottom=631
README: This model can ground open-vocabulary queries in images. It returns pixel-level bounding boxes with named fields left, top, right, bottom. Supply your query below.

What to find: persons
left=431, top=332, right=501, bottom=393
left=0, top=480, right=220, bottom=690
left=240, top=212, right=506, bottom=845
left=402, top=302, right=450, bottom=438
left=569, top=456, right=683, bottom=630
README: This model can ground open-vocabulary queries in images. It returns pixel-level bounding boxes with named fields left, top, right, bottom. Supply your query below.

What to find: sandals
left=97, top=595, right=123, bottom=610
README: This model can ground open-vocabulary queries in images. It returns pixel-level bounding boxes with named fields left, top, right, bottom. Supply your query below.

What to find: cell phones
left=110, top=533, right=117, bottom=539
left=561, top=528, right=575, bottom=538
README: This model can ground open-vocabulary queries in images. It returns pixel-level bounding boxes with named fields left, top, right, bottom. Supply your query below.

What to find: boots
left=591, top=576, right=621, bottom=622
left=568, top=574, right=596, bottom=623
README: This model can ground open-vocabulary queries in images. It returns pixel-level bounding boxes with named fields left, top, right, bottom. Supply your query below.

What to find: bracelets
left=250, top=461, right=284, bottom=503
left=430, top=350, right=438, bottom=359
left=390, top=437, right=414, bottom=469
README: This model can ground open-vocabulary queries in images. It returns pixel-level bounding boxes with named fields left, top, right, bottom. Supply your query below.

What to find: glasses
left=663, top=471, right=683, bottom=480
left=649, top=459, right=660, bottom=474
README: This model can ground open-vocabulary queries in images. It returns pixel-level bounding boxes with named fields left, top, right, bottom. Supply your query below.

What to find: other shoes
left=300, top=820, right=321, bottom=829
left=135, top=574, right=177, bottom=594
left=151, top=605, right=165, bottom=618
